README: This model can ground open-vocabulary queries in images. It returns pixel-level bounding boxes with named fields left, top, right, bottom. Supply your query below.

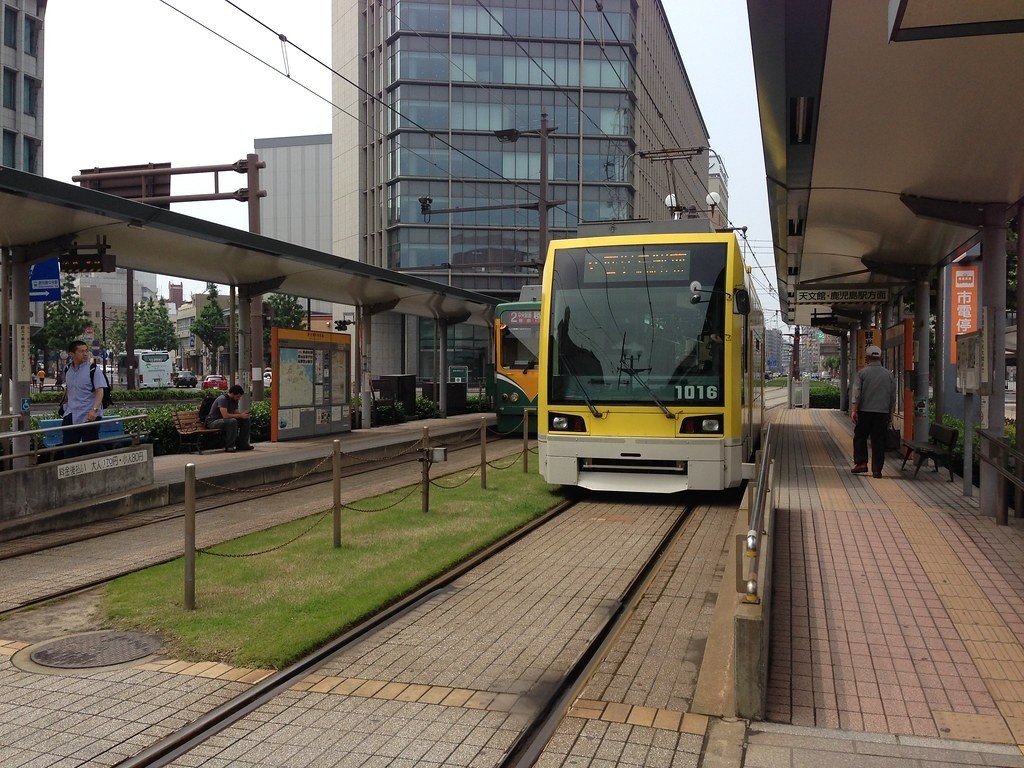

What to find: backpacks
left=198, top=394, right=231, bottom=422
left=63, top=361, right=115, bottom=410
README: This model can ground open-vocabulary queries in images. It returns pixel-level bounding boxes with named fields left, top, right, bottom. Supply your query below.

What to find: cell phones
left=246, top=411, right=252, bottom=414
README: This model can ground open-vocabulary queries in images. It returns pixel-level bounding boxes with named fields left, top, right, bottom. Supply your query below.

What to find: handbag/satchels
left=886, top=417, right=901, bottom=451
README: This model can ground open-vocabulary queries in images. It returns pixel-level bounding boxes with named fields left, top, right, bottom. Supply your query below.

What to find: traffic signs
left=29, top=257, right=63, bottom=302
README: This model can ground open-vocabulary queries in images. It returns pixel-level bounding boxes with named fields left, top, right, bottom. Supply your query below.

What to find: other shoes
left=236, top=445, right=255, bottom=450
left=851, top=462, right=868, bottom=473
left=224, top=447, right=237, bottom=452
left=873, top=471, right=883, bottom=479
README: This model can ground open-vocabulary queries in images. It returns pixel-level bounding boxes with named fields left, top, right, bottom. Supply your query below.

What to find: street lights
left=493, top=103, right=549, bottom=281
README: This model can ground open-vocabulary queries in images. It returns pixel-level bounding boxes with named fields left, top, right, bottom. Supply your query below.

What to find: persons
left=38, top=368, right=46, bottom=386
left=58, top=340, right=108, bottom=458
left=612, top=325, right=644, bottom=363
left=56, top=371, right=64, bottom=386
left=205, top=385, right=255, bottom=453
left=851, top=346, right=896, bottom=479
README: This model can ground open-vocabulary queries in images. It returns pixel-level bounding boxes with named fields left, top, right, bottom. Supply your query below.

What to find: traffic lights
left=334, top=320, right=351, bottom=325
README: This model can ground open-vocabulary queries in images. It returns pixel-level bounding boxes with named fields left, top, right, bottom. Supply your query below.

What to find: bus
left=117, top=349, right=174, bottom=390
left=493, top=283, right=542, bottom=434
left=538, top=147, right=764, bottom=496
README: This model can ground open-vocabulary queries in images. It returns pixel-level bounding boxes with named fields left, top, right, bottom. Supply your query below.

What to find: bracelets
left=93, top=408, right=98, bottom=411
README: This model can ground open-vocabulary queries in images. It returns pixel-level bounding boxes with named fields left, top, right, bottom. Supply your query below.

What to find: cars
left=173, top=371, right=197, bottom=388
left=263, top=372, right=272, bottom=387
left=95, top=364, right=118, bottom=374
left=764, top=370, right=832, bottom=381
left=202, top=374, right=228, bottom=390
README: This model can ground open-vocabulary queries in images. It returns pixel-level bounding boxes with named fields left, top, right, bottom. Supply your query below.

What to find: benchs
left=39, top=415, right=145, bottom=461
left=901, top=423, right=958, bottom=482
left=171, top=410, right=220, bottom=454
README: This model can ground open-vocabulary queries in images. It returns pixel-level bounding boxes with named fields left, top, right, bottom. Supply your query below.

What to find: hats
left=865, top=346, right=882, bottom=357
